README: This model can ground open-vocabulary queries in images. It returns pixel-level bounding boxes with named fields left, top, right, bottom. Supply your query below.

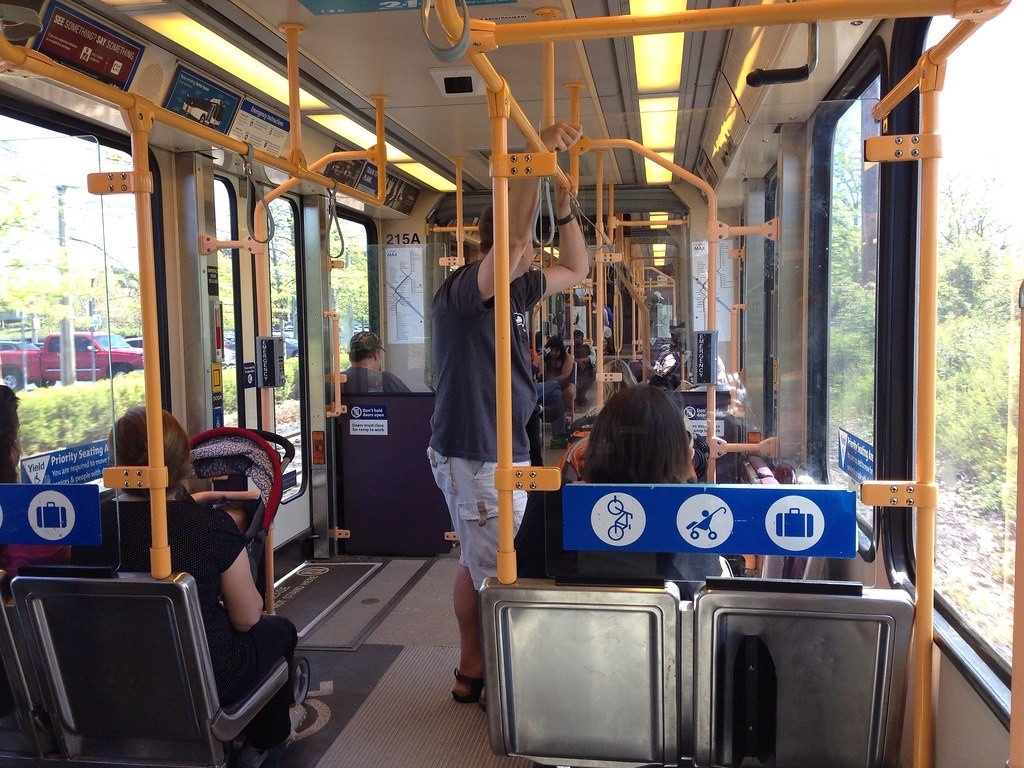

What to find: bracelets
left=555, top=212, right=575, bottom=225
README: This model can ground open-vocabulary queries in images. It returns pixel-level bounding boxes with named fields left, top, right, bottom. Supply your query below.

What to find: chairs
left=692, top=575, right=914, bottom=768
left=686, top=415, right=738, bottom=484
left=11, top=569, right=290, bottom=768
left=474, top=578, right=682, bottom=768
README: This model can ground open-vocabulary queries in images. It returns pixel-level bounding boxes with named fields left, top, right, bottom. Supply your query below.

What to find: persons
left=0, top=385, right=24, bottom=485
left=522, top=293, right=618, bottom=467
left=335, top=331, right=414, bottom=394
left=426, top=119, right=589, bottom=712
left=67, top=405, right=309, bottom=767
left=555, top=382, right=736, bottom=587
left=567, top=359, right=640, bottom=443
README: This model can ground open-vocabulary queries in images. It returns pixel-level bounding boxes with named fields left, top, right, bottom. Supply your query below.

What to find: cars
left=0, top=337, right=45, bottom=351
left=125, top=321, right=370, bottom=366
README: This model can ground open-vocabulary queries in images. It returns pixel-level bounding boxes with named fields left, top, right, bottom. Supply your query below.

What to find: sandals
left=451, top=667, right=487, bottom=711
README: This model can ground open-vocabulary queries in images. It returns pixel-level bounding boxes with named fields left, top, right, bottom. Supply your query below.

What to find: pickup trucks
left=0, top=332, right=144, bottom=389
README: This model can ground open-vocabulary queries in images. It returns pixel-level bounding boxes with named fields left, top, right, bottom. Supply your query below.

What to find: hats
left=349, top=331, right=383, bottom=352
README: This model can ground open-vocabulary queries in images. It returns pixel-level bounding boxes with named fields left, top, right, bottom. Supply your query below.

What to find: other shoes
left=548, top=434, right=576, bottom=449
left=280, top=701, right=308, bottom=751
